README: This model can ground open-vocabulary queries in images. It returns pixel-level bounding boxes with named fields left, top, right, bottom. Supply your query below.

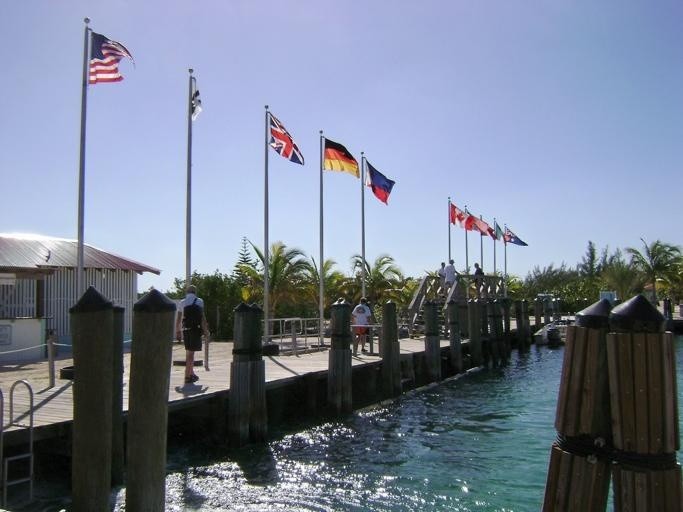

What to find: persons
left=174, top=285, right=211, bottom=383
left=352, top=296, right=373, bottom=355
left=471, top=262, right=485, bottom=300
left=437, top=262, right=447, bottom=300
left=442, top=258, right=460, bottom=299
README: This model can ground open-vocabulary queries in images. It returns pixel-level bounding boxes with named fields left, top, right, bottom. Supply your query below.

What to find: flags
left=465, top=210, right=488, bottom=236
left=481, top=220, right=498, bottom=242
left=265, top=111, right=306, bottom=167
left=320, top=139, right=360, bottom=181
left=450, top=203, right=475, bottom=231
left=505, top=227, right=528, bottom=246
left=190, top=76, right=204, bottom=125
left=87, top=32, right=138, bottom=84
left=364, top=160, right=396, bottom=208
left=495, top=224, right=506, bottom=247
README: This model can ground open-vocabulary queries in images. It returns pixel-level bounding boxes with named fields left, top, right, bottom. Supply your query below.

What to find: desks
left=349, top=324, right=385, bottom=356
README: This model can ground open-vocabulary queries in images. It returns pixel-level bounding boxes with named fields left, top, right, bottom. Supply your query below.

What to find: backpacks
left=182, top=301, right=204, bottom=330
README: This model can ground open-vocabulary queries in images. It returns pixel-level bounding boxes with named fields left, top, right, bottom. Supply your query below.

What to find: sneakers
left=191, top=374, right=199, bottom=382
left=184, top=376, right=194, bottom=383
